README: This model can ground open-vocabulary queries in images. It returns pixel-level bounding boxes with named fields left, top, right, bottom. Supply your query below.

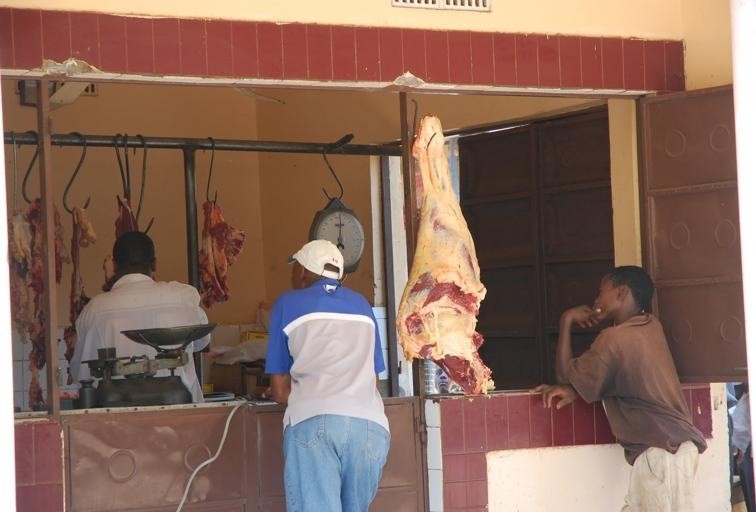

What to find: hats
left=288, top=239, right=344, bottom=280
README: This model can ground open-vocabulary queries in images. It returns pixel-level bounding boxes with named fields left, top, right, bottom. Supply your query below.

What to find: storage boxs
left=242, top=367, right=270, bottom=395
left=213, top=362, right=245, bottom=391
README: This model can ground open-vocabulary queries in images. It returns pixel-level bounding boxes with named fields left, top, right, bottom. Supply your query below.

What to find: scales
left=308, top=198, right=366, bottom=287
left=81, top=324, right=216, bottom=407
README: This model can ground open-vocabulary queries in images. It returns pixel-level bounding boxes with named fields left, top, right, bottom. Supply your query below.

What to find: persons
left=725, top=381, right=756, bottom=512
left=262, top=239, right=390, bottom=512
left=69, top=231, right=211, bottom=406
left=529, top=265, right=707, bottom=512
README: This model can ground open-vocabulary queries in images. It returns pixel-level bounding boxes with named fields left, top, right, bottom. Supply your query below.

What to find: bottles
left=67, top=367, right=72, bottom=384
left=255, top=304, right=268, bottom=333
left=57, top=367, right=63, bottom=386
